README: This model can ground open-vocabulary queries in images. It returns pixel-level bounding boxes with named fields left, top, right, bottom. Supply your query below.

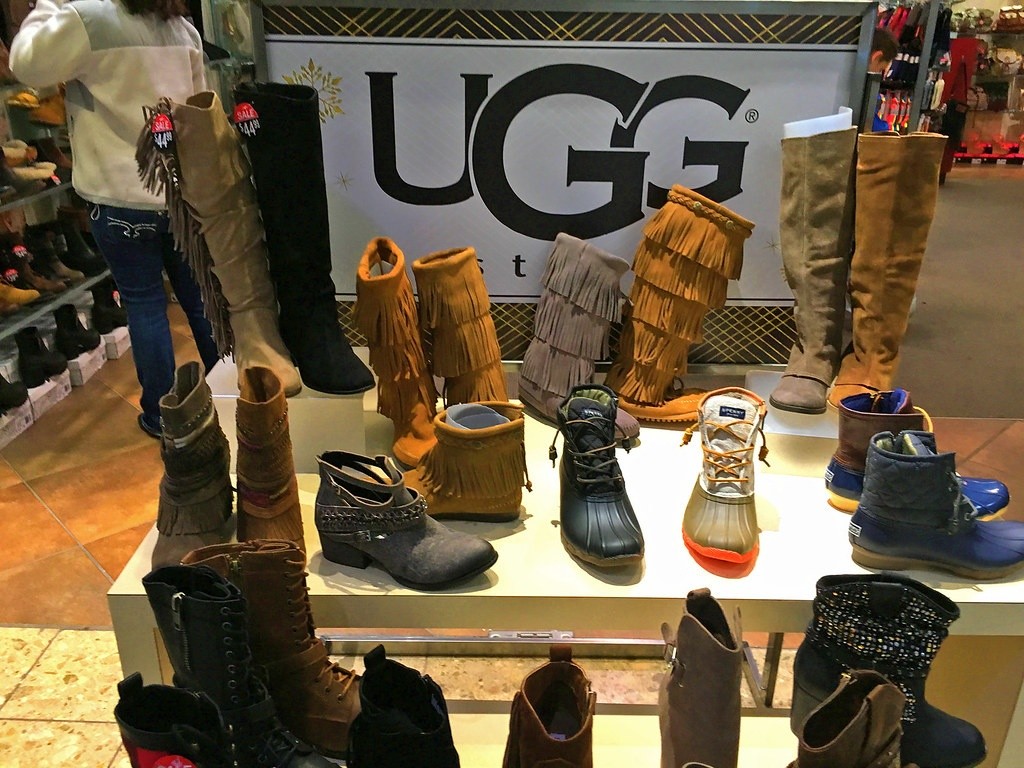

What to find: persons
left=8, top=0, right=220, bottom=440
left=868, top=27, right=898, bottom=132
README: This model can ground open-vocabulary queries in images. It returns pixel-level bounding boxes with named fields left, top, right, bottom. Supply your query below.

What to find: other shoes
left=137, top=412, right=162, bottom=438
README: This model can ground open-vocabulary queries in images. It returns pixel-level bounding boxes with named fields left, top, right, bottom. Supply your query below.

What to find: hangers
left=880, top=88, right=912, bottom=103
left=895, top=0, right=952, bottom=13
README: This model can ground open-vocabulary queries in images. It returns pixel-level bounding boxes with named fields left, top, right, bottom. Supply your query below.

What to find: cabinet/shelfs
left=0, top=183, right=111, bottom=343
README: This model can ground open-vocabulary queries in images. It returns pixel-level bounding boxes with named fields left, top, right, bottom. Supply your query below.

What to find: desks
left=106, top=398, right=1024, bottom=768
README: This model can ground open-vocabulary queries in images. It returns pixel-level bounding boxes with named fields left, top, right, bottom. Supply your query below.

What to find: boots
left=825, top=388, right=1024, bottom=580
left=399, top=401, right=529, bottom=521
left=355, top=234, right=438, bottom=466
left=825, top=132, right=948, bottom=413
left=235, top=82, right=376, bottom=397
left=409, top=244, right=509, bottom=406
left=0, top=94, right=128, bottom=413
left=149, top=363, right=306, bottom=578
left=679, top=386, right=772, bottom=565
left=520, top=232, right=642, bottom=443
left=767, top=107, right=859, bottom=414
left=133, top=92, right=304, bottom=399
left=313, top=448, right=500, bottom=592
left=607, top=185, right=755, bottom=422
left=548, top=384, right=646, bottom=567
left=113, top=542, right=985, bottom=768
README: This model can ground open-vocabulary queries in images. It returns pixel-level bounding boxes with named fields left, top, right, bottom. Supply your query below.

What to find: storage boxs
left=0, top=292, right=131, bottom=452
